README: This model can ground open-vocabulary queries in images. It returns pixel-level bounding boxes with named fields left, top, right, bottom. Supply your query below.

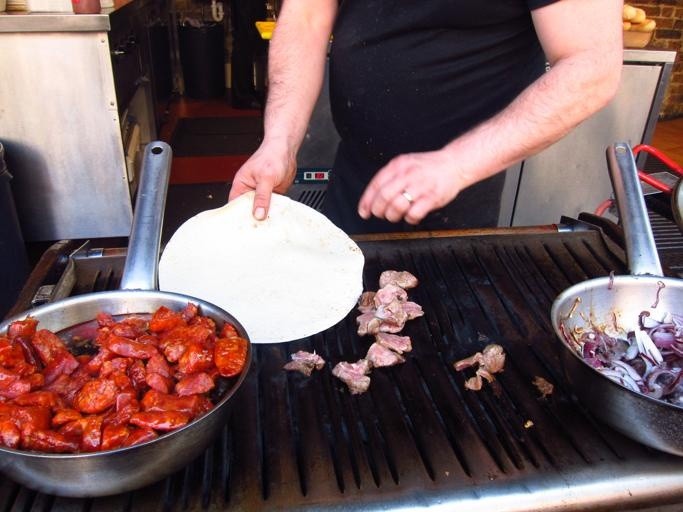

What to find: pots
left=1, top=141, right=255, bottom=496
left=549, top=144, right=681, bottom=455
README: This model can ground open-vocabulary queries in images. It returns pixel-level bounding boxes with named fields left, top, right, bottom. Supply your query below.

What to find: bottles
left=72, top=0, right=102, bottom=15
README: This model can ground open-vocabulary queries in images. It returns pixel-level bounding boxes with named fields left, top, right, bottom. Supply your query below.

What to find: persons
left=228, top=0, right=625, bottom=234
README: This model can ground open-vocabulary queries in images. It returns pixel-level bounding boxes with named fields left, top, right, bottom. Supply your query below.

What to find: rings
left=402, top=191, right=414, bottom=206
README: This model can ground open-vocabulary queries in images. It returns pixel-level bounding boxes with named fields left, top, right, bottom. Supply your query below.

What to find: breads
left=623, top=5, right=656, bottom=33
